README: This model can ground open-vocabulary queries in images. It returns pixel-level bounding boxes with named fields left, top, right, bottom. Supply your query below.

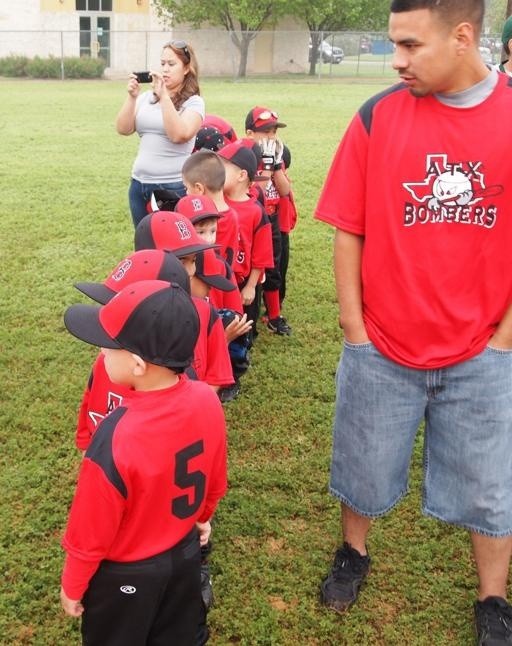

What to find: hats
left=218, top=138, right=264, bottom=182
left=194, top=127, right=224, bottom=153
left=63, top=279, right=200, bottom=367
left=193, top=238, right=237, bottom=292
left=134, top=194, right=225, bottom=258
left=73, top=247, right=192, bottom=306
left=246, top=106, right=288, bottom=130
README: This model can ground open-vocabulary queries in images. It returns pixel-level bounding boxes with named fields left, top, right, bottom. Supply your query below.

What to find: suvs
left=308, top=37, right=343, bottom=64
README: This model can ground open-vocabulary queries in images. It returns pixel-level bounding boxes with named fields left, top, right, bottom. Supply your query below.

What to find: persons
left=494, top=14, right=512, bottom=76
left=311, top=1, right=511, bottom=646
left=60, top=43, right=298, bottom=645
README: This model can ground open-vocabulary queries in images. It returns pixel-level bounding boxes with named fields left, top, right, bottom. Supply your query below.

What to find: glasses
left=161, top=39, right=191, bottom=61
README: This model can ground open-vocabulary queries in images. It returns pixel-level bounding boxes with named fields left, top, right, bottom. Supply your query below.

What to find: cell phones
left=132, top=72, right=152, bottom=83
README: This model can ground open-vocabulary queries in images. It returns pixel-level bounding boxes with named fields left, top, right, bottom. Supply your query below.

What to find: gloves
left=259, top=137, right=284, bottom=171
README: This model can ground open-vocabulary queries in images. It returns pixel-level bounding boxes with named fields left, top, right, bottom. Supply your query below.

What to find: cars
left=359, top=35, right=373, bottom=53
left=480, top=31, right=502, bottom=67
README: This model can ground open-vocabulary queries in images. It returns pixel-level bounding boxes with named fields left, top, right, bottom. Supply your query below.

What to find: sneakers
left=320, top=541, right=372, bottom=611
left=473, top=595, right=511, bottom=645
left=199, top=563, right=213, bottom=611
left=218, top=378, right=242, bottom=404
left=262, top=307, right=291, bottom=335
